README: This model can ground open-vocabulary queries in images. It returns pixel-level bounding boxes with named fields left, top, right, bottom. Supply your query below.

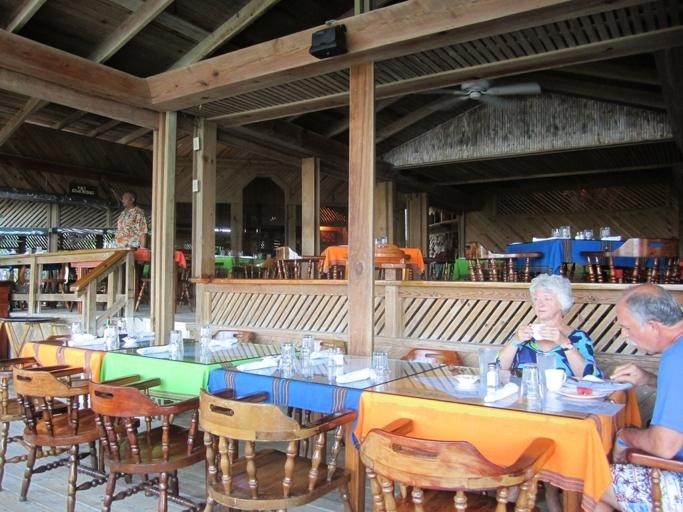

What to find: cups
left=379, top=236, right=387, bottom=245
left=519, top=362, right=544, bottom=398
left=498, top=370, right=517, bottom=388
left=479, top=348, right=496, bottom=376
left=71, top=322, right=81, bottom=334
left=169, top=330, right=184, bottom=355
left=280, top=336, right=344, bottom=367
left=544, top=369, right=567, bottom=391
left=551, top=225, right=611, bottom=240
left=104, top=325, right=119, bottom=350
left=198, top=321, right=212, bottom=347
left=373, top=352, right=389, bottom=372
left=529, top=324, right=546, bottom=341
left=127, top=328, right=136, bottom=339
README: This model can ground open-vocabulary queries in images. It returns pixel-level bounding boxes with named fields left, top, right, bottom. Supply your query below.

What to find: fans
left=419, top=79, right=542, bottom=112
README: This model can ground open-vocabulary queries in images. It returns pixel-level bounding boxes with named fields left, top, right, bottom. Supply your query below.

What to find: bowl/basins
left=455, top=373, right=479, bottom=384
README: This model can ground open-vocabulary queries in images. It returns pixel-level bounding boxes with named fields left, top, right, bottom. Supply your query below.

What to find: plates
left=559, top=387, right=606, bottom=398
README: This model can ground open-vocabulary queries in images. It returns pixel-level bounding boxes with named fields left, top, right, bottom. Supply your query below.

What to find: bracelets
left=614, top=425, right=638, bottom=448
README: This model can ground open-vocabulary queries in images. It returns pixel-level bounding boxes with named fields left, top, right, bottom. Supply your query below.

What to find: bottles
left=485, top=363, right=498, bottom=401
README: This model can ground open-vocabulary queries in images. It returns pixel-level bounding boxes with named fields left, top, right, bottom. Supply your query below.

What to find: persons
left=581, top=282, right=682, bottom=511
left=115, top=188, right=148, bottom=309
left=495, top=273, right=607, bottom=503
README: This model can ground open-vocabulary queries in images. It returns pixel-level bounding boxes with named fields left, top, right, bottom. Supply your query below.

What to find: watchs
left=561, top=341, right=573, bottom=352
